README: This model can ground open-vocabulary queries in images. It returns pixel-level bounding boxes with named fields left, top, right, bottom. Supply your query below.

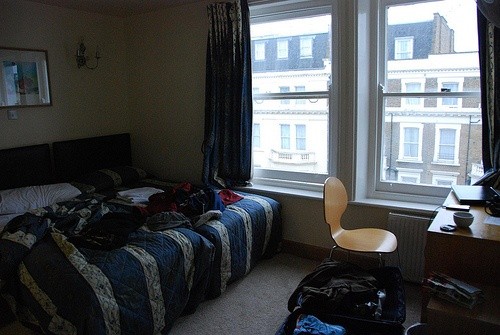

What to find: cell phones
left=446, top=204, right=470, bottom=211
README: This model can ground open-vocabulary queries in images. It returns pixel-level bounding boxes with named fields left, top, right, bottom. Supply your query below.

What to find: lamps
left=76, top=40, right=102, bottom=70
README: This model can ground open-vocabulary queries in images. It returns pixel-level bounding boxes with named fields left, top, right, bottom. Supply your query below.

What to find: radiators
left=384, top=212, right=435, bottom=284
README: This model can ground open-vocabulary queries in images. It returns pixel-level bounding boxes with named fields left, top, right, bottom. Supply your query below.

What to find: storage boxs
left=425, top=277, right=500, bottom=335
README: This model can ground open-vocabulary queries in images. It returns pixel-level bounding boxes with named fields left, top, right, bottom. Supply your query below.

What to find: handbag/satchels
left=172, top=180, right=225, bottom=215
left=69, top=208, right=146, bottom=252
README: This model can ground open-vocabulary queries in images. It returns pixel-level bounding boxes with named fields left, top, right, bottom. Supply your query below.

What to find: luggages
left=274, top=257, right=407, bottom=334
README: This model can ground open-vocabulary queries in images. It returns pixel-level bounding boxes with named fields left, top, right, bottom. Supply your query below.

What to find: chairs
left=323, top=176, right=397, bottom=268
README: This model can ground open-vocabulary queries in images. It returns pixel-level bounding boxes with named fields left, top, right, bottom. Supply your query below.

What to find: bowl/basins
left=453, top=212, right=474, bottom=227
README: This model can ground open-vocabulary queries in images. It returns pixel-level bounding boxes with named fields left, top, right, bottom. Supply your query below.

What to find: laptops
left=451, top=185, right=500, bottom=206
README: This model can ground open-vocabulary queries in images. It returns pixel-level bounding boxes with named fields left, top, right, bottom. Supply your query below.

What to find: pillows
left=91, top=166, right=147, bottom=186
left=0, top=183, right=82, bottom=216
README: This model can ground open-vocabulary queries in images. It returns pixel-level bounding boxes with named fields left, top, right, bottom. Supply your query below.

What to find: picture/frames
left=0, top=47, right=52, bottom=109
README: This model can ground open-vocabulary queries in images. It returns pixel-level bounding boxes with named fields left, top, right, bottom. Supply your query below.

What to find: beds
left=0, top=133, right=281, bottom=335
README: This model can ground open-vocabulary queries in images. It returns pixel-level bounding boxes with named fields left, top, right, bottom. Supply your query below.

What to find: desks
left=420, top=184, right=500, bottom=323
